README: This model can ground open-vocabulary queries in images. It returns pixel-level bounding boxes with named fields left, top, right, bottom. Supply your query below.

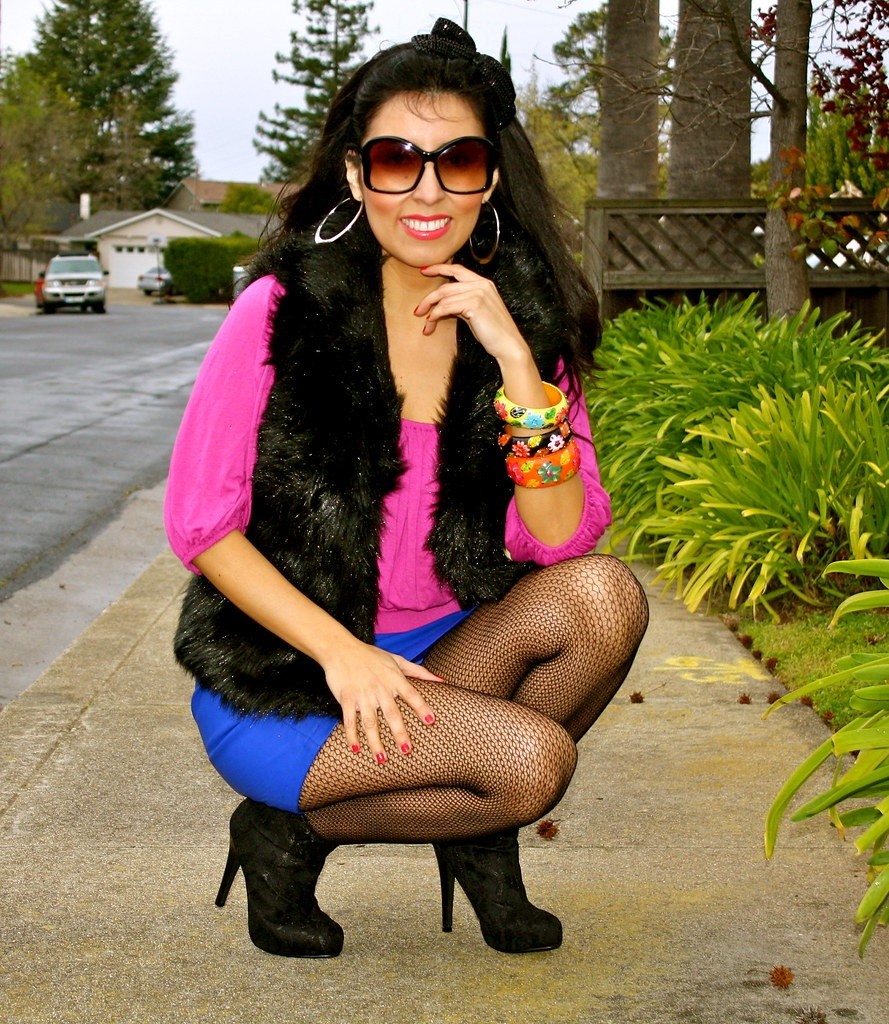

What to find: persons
left=165, top=16, right=654, bottom=961
left=34, top=271, right=46, bottom=307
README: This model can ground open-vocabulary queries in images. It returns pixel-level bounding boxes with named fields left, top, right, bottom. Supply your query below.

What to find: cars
left=38, top=250, right=109, bottom=314
left=137, top=265, right=183, bottom=296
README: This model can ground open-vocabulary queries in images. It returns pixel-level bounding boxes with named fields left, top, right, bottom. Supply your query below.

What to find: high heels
left=431, top=827, right=563, bottom=954
left=212, top=795, right=346, bottom=960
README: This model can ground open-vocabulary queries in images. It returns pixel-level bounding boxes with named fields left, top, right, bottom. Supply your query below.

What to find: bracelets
left=492, top=380, right=571, bottom=431
left=496, top=417, right=573, bottom=458
left=503, top=441, right=582, bottom=489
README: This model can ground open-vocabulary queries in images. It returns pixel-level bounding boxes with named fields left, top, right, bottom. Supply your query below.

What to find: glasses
left=345, top=134, right=504, bottom=196
